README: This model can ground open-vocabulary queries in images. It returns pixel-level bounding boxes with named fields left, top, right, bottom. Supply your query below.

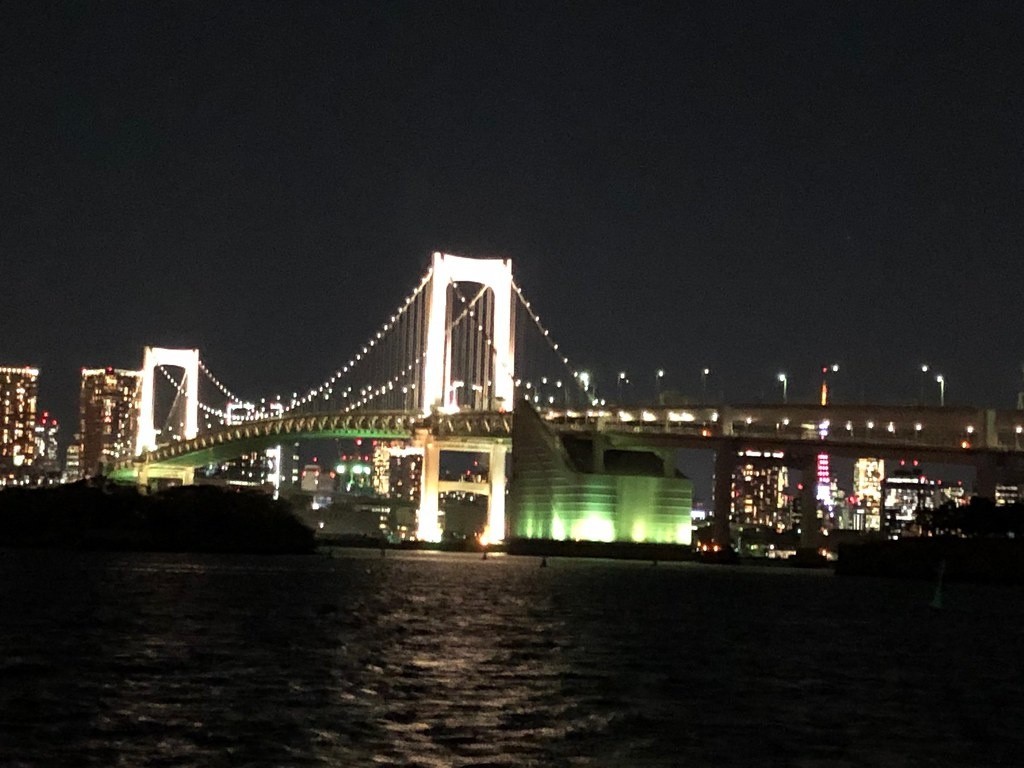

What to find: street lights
left=699, top=368, right=709, bottom=406
left=936, top=375, right=945, bottom=405
left=778, top=373, right=787, bottom=406
left=656, top=368, right=665, bottom=411
